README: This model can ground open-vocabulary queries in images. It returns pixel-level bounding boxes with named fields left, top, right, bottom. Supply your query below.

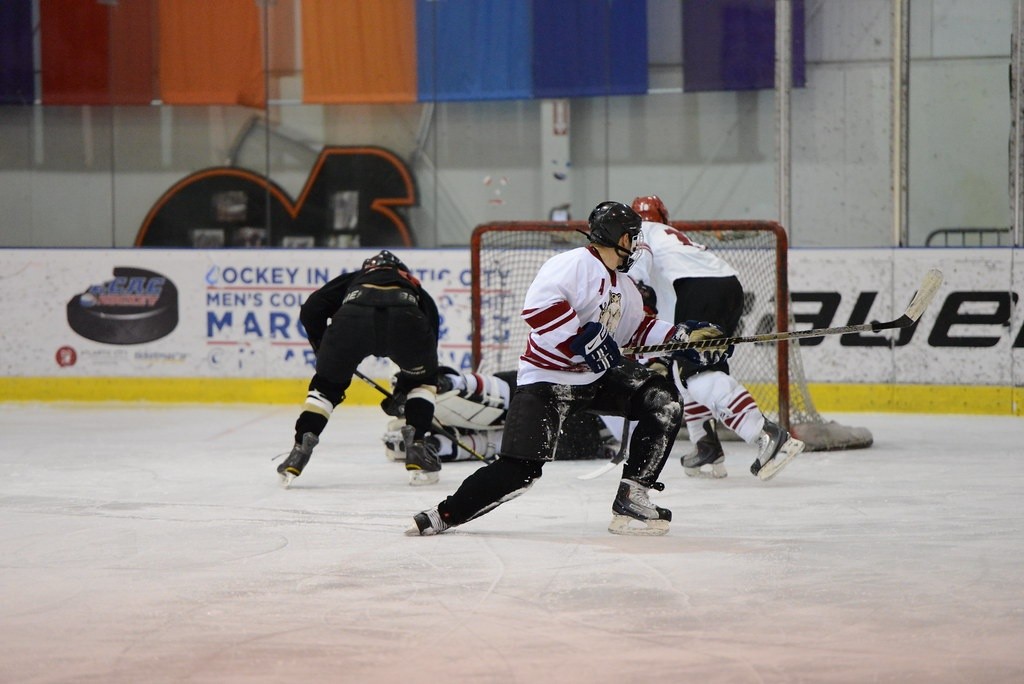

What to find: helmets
left=631, top=196, right=669, bottom=226
left=588, top=202, right=642, bottom=273
left=361, top=250, right=409, bottom=273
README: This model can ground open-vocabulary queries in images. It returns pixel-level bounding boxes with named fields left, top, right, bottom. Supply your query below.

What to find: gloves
left=669, top=320, right=735, bottom=365
left=568, top=321, right=622, bottom=373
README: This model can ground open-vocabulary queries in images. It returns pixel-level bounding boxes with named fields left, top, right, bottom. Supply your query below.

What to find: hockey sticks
left=352, top=370, right=492, bottom=465
left=591, top=267, right=942, bottom=357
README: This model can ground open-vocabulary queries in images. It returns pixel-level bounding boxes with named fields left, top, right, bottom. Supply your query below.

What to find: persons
left=266, top=250, right=443, bottom=489
left=381, top=196, right=805, bottom=546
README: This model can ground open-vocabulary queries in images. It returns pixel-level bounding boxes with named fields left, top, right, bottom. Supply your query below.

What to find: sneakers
left=750, top=414, right=805, bottom=481
left=608, top=463, right=672, bottom=535
left=401, top=425, right=442, bottom=485
left=680, top=417, right=728, bottom=478
left=278, top=433, right=319, bottom=489
left=405, top=504, right=451, bottom=536
